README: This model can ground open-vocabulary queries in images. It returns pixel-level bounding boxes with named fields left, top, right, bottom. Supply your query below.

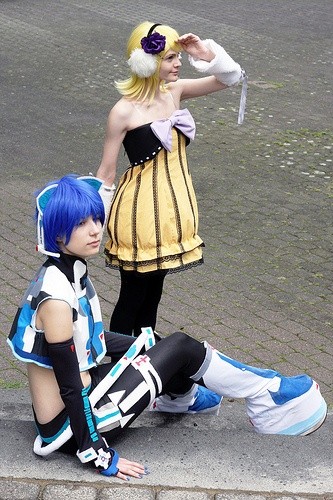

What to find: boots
left=193, top=341, right=327, bottom=436
left=149, top=381, right=221, bottom=414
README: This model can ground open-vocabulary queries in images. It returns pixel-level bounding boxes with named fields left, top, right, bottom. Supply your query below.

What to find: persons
left=5, top=173, right=327, bottom=483
left=97, top=18, right=249, bottom=345
